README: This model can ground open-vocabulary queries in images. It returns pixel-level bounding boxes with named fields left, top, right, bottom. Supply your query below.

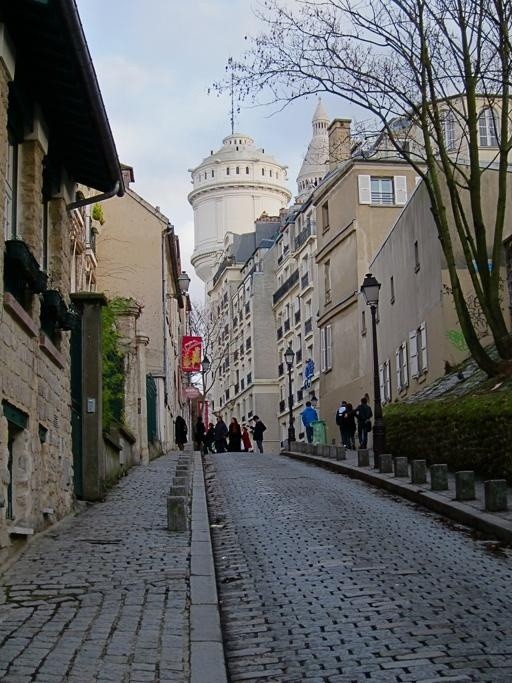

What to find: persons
left=302, top=402, right=318, bottom=443
left=354, top=398, right=372, bottom=449
left=342, top=404, right=359, bottom=450
left=250, top=415, right=266, bottom=453
left=241, top=427, right=252, bottom=452
left=194, top=417, right=205, bottom=451
left=175, top=416, right=187, bottom=450
left=336, top=401, right=352, bottom=448
left=214, top=416, right=228, bottom=453
left=226, top=418, right=242, bottom=452
left=203, top=423, right=215, bottom=454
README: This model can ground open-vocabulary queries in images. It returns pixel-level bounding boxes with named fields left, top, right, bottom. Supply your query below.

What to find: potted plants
left=91, top=202, right=106, bottom=235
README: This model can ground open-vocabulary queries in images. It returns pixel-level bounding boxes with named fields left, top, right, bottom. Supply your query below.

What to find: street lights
left=357, top=271, right=390, bottom=470
left=185, top=356, right=212, bottom=446
left=283, top=346, right=297, bottom=451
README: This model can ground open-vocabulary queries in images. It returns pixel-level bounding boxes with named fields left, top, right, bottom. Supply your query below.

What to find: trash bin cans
left=310, top=419, right=327, bottom=446
